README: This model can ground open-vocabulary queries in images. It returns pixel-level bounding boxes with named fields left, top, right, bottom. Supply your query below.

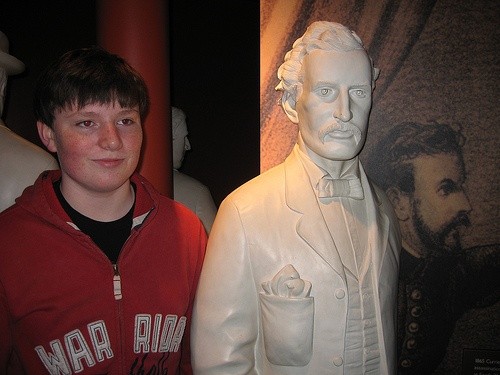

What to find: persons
left=170, top=107, right=218, bottom=235
left=192, top=19, right=400, bottom=374
left=0, top=47, right=209, bottom=375
left=2, top=37, right=61, bottom=210
